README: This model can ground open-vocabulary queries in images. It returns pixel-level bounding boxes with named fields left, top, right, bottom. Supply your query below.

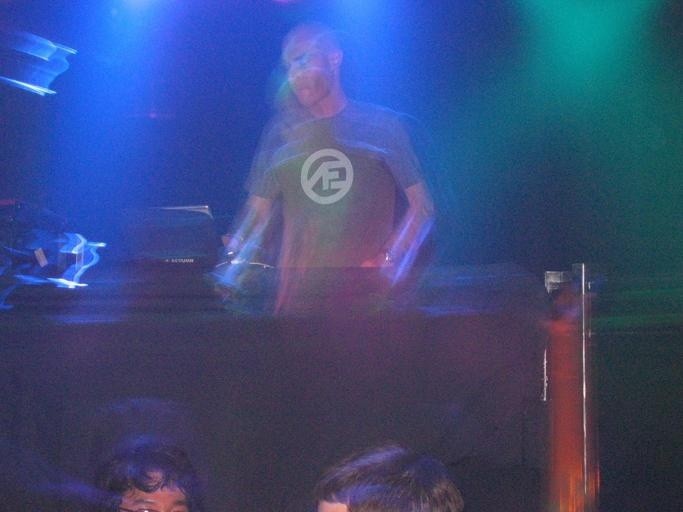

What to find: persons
left=99, top=443, right=199, bottom=512
left=315, top=443, right=466, bottom=512
left=216, top=21, right=437, bottom=310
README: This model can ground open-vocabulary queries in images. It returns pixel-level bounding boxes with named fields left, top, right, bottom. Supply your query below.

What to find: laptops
left=115, top=205, right=275, bottom=273
